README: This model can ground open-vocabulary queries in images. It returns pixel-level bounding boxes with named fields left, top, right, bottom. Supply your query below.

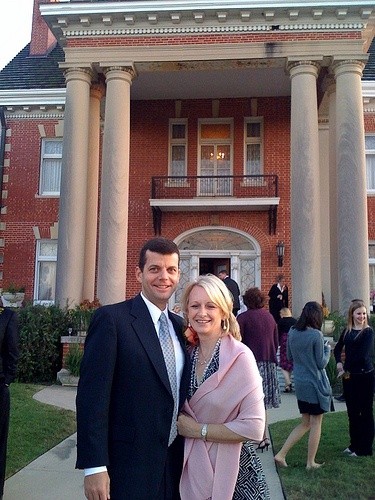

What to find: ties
left=158, top=312, right=178, bottom=447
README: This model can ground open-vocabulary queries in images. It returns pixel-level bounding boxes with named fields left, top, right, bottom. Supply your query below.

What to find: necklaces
left=191, top=337, right=221, bottom=389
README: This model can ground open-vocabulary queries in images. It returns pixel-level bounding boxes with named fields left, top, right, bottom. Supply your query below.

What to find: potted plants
left=57, top=341, right=84, bottom=388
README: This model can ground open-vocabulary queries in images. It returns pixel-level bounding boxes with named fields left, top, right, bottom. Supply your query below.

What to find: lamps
left=277, top=240, right=285, bottom=267
left=2, top=286, right=27, bottom=309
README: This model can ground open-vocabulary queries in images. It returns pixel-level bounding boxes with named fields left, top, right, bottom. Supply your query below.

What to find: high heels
left=264, top=437, right=271, bottom=451
left=253, top=440, right=265, bottom=453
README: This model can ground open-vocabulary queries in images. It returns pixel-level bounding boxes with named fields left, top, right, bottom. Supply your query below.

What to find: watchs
left=201, top=423, right=208, bottom=441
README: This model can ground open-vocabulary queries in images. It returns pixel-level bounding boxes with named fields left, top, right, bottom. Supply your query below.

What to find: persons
left=278, top=307, right=299, bottom=393
left=219, top=270, right=241, bottom=317
left=335, top=300, right=375, bottom=457
left=268, top=275, right=289, bottom=323
left=275, top=300, right=331, bottom=469
left=77, top=237, right=191, bottom=499
left=177, top=274, right=275, bottom=500
left=235, top=286, right=282, bottom=452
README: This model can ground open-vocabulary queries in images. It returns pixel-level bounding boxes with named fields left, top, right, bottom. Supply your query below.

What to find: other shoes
left=283, top=383, right=291, bottom=392
left=344, top=448, right=357, bottom=456
